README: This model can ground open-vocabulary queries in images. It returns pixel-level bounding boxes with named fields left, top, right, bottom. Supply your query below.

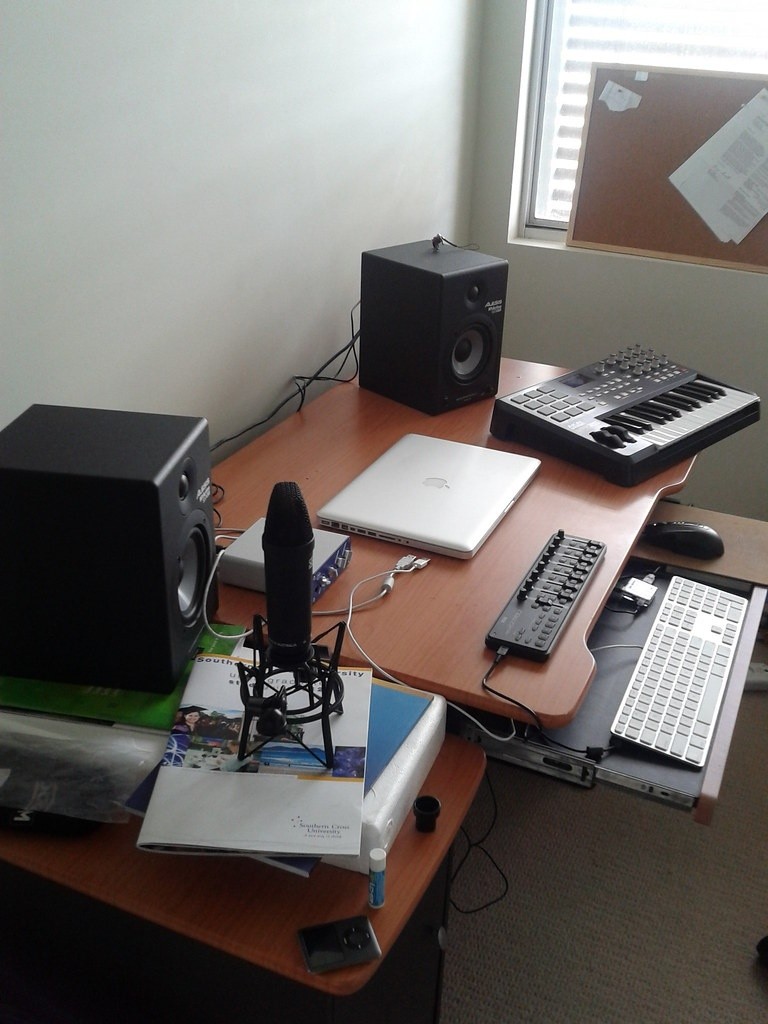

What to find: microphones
left=260, top=481, right=315, bottom=662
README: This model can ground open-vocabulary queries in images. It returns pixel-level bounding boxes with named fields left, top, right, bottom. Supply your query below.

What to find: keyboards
left=611, top=577, right=748, bottom=767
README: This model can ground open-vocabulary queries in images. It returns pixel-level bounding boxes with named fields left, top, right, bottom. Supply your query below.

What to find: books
left=136, top=652, right=374, bottom=859
left=1, top=625, right=247, bottom=737
left=123, top=676, right=434, bottom=880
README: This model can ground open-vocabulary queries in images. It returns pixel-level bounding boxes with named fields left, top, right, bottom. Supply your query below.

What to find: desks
left=0, top=334, right=710, bottom=1017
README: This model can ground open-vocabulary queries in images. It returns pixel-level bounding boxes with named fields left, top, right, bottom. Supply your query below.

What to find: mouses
left=653, top=521, right=725, bottom=561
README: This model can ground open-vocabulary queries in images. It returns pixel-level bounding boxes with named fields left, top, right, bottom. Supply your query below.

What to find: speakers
left=359, top=240, right=508, bottom=416
left=0, top=404, right=219, bottom=695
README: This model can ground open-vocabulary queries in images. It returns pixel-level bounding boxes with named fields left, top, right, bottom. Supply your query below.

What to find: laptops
left=316, top=432, right=542, bottom=563
